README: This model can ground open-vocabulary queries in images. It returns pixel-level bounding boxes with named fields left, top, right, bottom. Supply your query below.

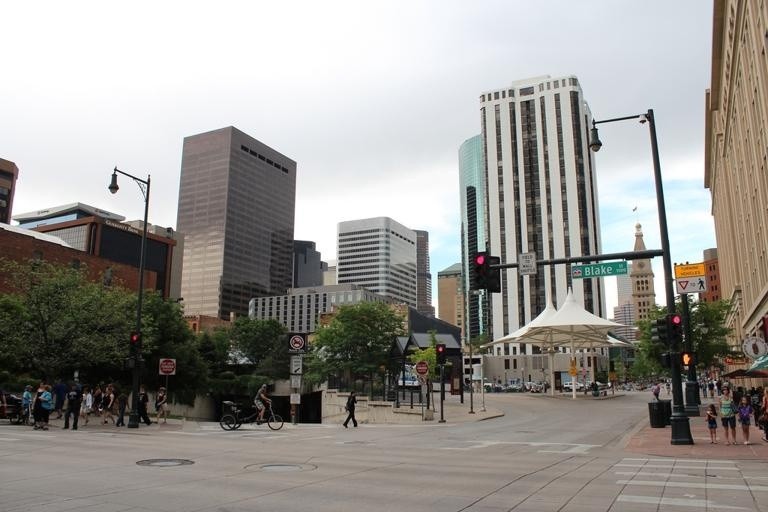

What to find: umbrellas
left=478, top=287, right=630, bottom=400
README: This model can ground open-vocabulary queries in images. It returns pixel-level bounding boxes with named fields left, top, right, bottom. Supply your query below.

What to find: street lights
left=106, top=164, right=153, bottom=430
left=587, top=106, right=695, bottom=447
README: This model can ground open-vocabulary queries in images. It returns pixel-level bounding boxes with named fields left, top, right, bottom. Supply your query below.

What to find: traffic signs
left=471, top=251, right=488, bottom=290
left=565, top=261, right=630, bottom=277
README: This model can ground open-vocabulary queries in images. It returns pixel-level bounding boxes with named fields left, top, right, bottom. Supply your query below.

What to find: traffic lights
left=128, top=330, right=143, bottom=360
left=667, top=312, right=683, bottom=354
left=655, top=315, right=669, bottom=347
left=433, top=342, right=448, bottom=366
left=680, top=351, right=693, bottom=366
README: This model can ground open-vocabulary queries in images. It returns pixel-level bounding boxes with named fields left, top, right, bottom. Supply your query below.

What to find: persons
left=254, top=384, right=272, bottom=422
left=699, top=377, right=768, bottom=446
left=651, top=382, right=672, bottom=400
left=0, top=377, right=168, bottom=430
left=343, top=391, right=357, bottom=428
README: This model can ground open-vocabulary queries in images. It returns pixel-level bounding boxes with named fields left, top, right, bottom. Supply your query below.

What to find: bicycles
left=9, top=400, right=36, bottom=426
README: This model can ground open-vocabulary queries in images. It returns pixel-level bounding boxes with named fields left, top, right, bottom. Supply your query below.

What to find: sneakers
left=709, top=437, right=768, bottom=445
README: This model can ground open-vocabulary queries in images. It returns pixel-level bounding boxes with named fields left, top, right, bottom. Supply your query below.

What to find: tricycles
left=218, top=398, right=284, bottom=431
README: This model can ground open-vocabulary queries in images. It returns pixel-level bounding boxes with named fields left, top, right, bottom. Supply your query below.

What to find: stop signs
left=414, top=361, right=428, bottom=375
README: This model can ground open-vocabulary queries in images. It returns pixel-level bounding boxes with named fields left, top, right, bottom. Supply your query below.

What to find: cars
left=480, top=379, right=608, bottom=393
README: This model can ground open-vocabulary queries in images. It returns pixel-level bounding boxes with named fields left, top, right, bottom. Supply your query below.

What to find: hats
left=25, top=384, right=33, bottom=390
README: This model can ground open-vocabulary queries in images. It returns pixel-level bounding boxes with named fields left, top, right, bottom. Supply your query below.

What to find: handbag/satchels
left=758, top=410, right=768, bottom=424
left=729, top=402, right=739, bottom=414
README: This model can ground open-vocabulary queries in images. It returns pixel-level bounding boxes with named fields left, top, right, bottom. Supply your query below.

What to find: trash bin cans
left=648, top=399, right=671, bottom=428
